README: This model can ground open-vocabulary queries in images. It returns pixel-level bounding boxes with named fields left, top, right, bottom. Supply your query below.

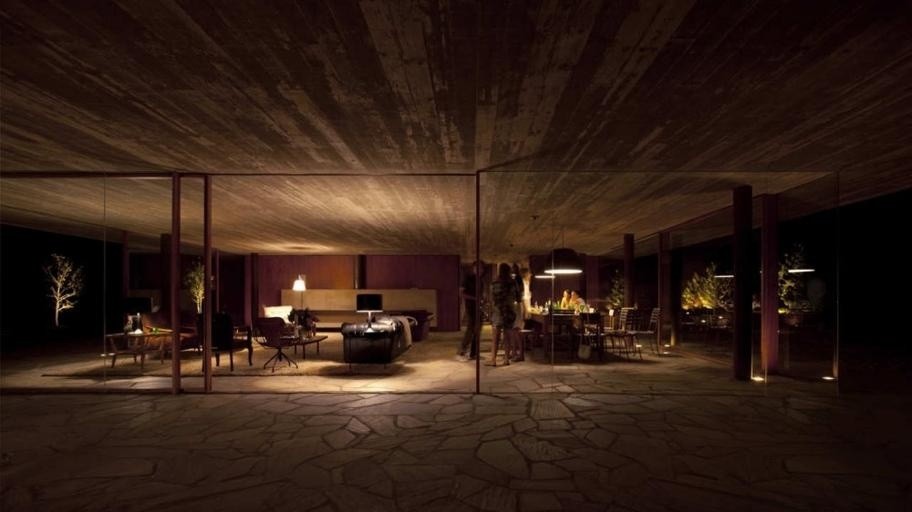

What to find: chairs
left=102, top=308, right=433, bottom=374
left=492, top=304, right=666, bottom=364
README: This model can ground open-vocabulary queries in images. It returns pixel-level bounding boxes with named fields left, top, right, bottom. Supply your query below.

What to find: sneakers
left=468, top=355, right=485, bottom=360
left=483, top=361, right=496, bottom=366
left=503, top=351, right=524, bottom=362
left=501, top=359, right=510, bottom=366
left=454, top=353, right=468, bottom=362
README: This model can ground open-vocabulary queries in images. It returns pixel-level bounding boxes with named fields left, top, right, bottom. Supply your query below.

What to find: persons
left=567, top=288, right=587, bottom=327
left=455, top=260, right=485, bottom=363
left=559, top=286, right=570, bottom=307
left=484, top=262, right=533, bottom=368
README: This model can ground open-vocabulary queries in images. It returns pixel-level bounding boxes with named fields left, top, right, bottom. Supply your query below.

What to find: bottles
left=533, top=300, right=560, bottom=314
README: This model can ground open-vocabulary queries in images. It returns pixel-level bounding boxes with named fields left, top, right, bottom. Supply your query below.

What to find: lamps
left=292, top=272, right=307, bottom=311
left=534, top=265, right=556, bottom=280
left=786, top=241, right=817, bottom=274
left=541, top=223, right=585, bottom=274
left=712, top=262, right=737, bottom=279
